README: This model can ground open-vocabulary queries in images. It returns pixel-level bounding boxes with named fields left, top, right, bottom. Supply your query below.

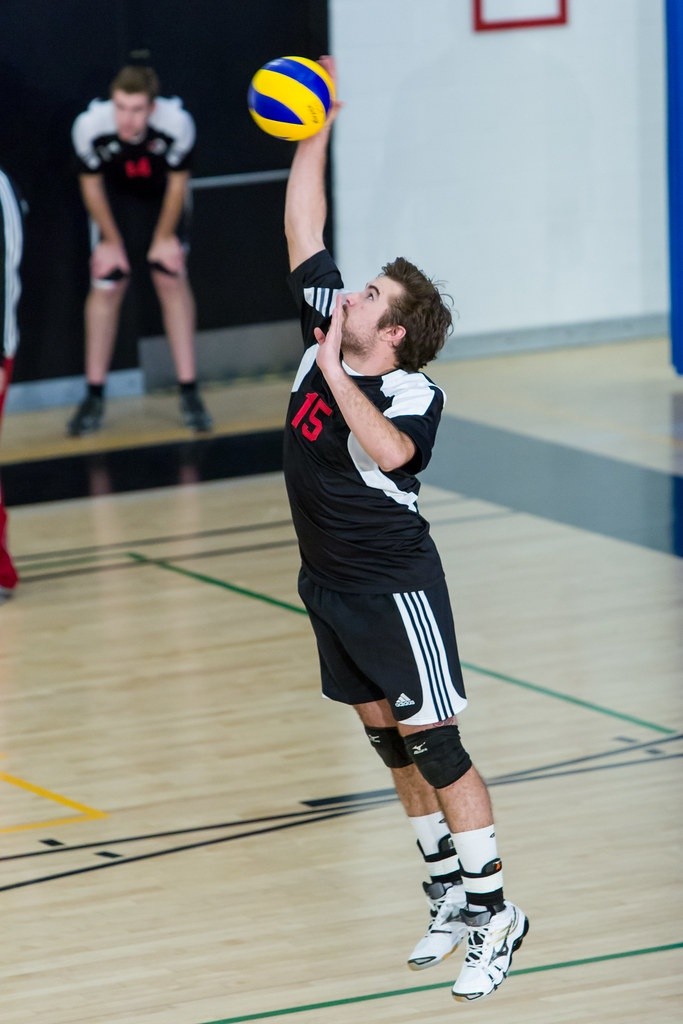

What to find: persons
left=0, top=170, right=24, bottom=606
left=281, top=55, right=530, bottom=1004
left=63, top=66, right=216, bottom=433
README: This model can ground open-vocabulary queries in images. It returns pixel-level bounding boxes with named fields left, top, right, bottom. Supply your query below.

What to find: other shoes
left=178, top=379, right=213, bottom=437
left=69, top=384, right=108, bottom=441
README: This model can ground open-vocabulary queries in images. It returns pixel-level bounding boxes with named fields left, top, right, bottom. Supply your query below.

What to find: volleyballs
left=247, top=54, right=337, bottom=142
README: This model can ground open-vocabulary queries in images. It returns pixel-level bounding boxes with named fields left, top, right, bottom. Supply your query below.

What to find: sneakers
left=409, top=884, right=469, bottom=970
left=451, top=902, right=528, bottom=999
